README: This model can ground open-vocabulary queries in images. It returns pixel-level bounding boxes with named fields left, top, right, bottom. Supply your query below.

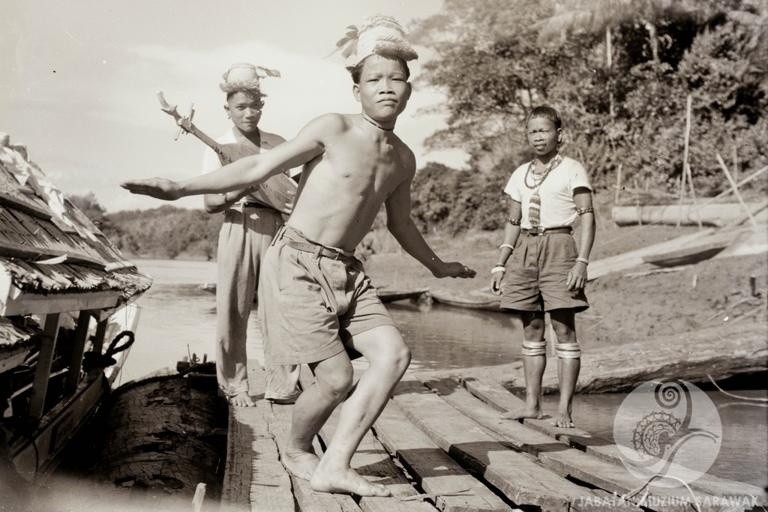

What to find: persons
left=489, top=106, right=594, bottom=428
left=112, top=14, right=476, bottom=496
left=200, top=59, right=297, bottom=410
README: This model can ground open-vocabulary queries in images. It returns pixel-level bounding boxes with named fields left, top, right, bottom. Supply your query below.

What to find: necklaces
left=363, top=116, right=396, bottom=132
left=524, top=155, right=555, bottom=188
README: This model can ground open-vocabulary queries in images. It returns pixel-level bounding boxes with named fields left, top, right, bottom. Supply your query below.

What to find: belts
left=281, top=225, right=358, bottom=266
left=520, top=226, right=572, bottom=235
left=232, top=202, right=272, bottom=209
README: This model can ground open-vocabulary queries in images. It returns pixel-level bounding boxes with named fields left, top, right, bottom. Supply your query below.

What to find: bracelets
left=496, top=244, right=514, bottom=250
left=573, top=257, right=591, bottom=264
left=490, top=262, right=509, bottom=275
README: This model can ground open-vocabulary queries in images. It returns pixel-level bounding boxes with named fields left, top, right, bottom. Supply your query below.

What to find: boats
left=1, top=128, right=156, bottom=511
left=376, top=287, right=430, bottom=305
left=427, top=285, right=510, bottom=313
left=201, top=281, right=216, bottom=297
